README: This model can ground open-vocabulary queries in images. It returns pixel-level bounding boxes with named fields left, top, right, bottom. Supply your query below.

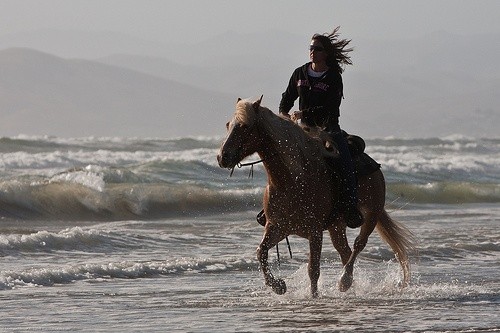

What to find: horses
left=216, top=94, right=420, bottom=300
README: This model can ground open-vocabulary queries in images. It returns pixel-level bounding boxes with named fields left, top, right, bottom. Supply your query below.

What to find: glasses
left=309, top=45, right=323, bottom=52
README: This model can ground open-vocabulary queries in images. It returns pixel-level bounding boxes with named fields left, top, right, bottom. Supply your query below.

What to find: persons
left=278, top=33, right=364, bottom=229
